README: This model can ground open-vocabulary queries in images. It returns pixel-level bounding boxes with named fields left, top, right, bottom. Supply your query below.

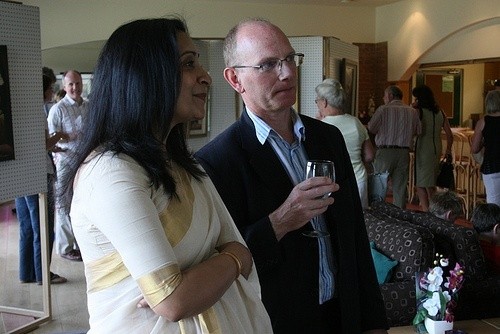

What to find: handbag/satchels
left=437, top=161, right=455, bottom=189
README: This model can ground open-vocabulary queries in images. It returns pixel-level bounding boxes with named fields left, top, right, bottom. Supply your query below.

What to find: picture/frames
left=236, top=53, right=299, bottom=120
left=0, top=45, right=16, bottom=162
left=190, top=87, right=210, bottom=138
left=341, top=58, right=358, bottom=116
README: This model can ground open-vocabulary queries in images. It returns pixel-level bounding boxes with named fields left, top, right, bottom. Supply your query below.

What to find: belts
left=380, top=145, right=400, bottom=149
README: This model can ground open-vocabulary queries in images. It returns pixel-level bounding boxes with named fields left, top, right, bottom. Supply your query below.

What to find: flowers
left=412, top=263, right=463, bottom=325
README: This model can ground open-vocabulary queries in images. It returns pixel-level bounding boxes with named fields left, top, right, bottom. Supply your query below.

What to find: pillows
left=370, top=241, right=398, bottom=285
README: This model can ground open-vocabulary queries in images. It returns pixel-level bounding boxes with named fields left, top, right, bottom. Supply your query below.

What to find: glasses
left=232, top=53, right=305, bottom=74
left=315, top=99, right=323, bottom=104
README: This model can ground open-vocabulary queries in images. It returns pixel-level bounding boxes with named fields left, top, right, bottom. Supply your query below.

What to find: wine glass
left=304, top=159, right=337, bottom=239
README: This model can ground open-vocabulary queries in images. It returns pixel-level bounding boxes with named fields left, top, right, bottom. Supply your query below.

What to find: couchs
left=363, top=200, right=500, bottom=326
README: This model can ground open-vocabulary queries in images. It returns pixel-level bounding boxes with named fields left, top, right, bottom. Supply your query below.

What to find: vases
left=424, top=317, right=453, bottom=334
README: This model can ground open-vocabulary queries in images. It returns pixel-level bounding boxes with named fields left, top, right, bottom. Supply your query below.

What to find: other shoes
left=61, top=249, right=81, bottom=260
left=37, top=271, right=66, bottom=284
left=20, top=277, right=35, bottom=282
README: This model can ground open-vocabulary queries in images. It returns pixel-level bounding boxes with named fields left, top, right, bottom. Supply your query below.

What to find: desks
left=441, top=128, right=485, bottom=197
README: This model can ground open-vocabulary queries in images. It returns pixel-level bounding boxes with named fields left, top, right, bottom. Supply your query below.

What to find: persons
left=412, top=85, right=454, bottom=213
left=429, top=191, right=464, bottom=223
left=194, top=18, right=389, bottom=334
left=470, top=90, right=500, bottom=207
left=312, top=78, right=375, bottom=211
left=65, top=16, right=274, bottom=334
left=365, top=85, right=418, bottom=210
left=472, top=203, right=500, bottom=275
left=7, top=66, right=69, bottom=285
left=47, top=69, right=92, bottom=262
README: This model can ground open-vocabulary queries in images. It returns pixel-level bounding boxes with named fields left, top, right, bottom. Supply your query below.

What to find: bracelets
left=207, top=250, right=243, bottom=280
